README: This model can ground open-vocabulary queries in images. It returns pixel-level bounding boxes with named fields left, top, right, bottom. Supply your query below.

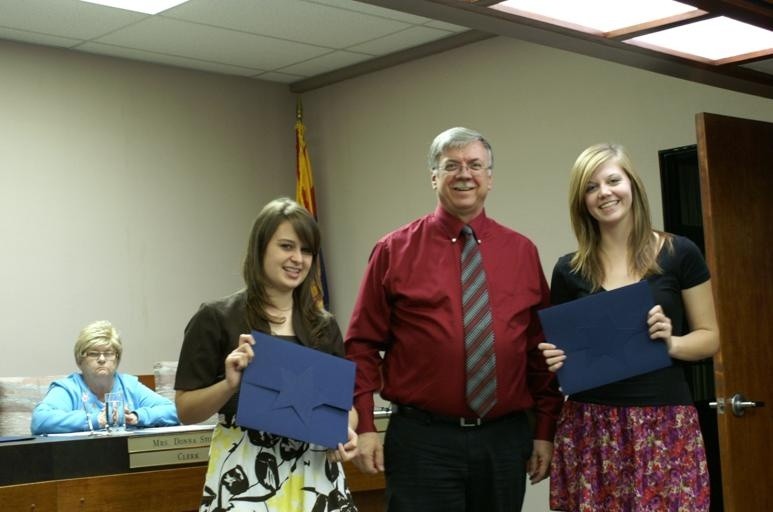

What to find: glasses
left=87, top=350, right=117, bottom=361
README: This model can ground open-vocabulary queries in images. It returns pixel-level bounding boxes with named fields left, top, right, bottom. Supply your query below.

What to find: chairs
left=1, top=374, right=66, bottom=438
left=153, top=360, right=219, bottom=429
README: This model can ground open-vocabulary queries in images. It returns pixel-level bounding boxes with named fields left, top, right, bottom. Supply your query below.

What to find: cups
left=103, top=392, right=125, bottom=434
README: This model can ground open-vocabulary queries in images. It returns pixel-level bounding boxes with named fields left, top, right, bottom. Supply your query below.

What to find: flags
left=294, top=122, right=329, bottom=313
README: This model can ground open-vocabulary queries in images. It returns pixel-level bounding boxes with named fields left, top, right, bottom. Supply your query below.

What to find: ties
left=460, top=224, right=499, bottom=420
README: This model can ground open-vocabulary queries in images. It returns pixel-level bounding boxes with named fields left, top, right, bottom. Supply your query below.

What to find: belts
left=396, top=402, right=535, bottom=430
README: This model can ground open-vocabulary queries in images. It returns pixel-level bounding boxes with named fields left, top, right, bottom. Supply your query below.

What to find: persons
left=173, top=196, right=359, bottom=512
left=538, top=143, right=720, bottom=512
left=30, top=320, right=180, bottom=434
left=339, top=126, right=562, bottom=512
